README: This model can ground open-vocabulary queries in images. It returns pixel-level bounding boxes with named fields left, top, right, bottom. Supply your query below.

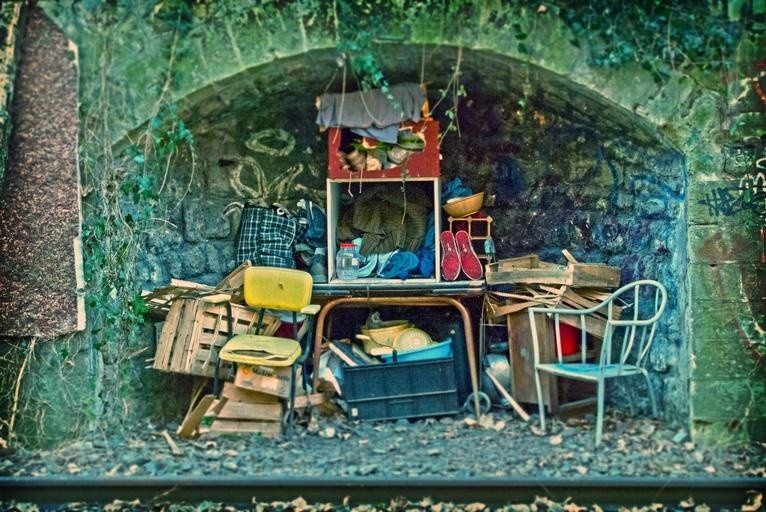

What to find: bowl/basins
left=381, top=337, right=452, bottom=365
left=442, top=190, right=485, bottom=218
left=393, top=327, right=433, bottom=353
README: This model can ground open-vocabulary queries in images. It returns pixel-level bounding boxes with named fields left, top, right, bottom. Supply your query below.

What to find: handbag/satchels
left=237, top=199, right=328, bottom=283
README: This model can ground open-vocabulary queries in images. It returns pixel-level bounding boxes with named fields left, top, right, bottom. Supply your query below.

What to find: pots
left=355, top=320, right=416, bottom=357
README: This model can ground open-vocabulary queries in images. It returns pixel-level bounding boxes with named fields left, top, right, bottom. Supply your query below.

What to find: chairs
left=528, top=279, right=668, bottom=447
left=211, top=265, right=314, bottom=441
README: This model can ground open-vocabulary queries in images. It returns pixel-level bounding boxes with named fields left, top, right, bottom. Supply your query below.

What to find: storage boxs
left=234, top=364, right=302, bottom=399
left=338, top=352, right=462, bottom=422
left=324, top=118, right=441, bottom=287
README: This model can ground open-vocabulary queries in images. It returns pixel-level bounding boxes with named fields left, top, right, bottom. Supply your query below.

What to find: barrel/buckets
left=554, top=318, right=580, bottom=355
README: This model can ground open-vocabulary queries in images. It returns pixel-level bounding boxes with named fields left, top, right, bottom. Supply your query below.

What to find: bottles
left=336, top=243, right=359, bottom=281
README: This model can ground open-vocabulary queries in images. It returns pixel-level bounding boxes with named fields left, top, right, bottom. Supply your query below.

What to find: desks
left=311, top=282, right=487, bottom=421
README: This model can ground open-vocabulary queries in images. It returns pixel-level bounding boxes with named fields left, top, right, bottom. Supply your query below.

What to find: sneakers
left=440, top=230, right=484, bottom=282
left=337, top=129, right=424, bottom=171
